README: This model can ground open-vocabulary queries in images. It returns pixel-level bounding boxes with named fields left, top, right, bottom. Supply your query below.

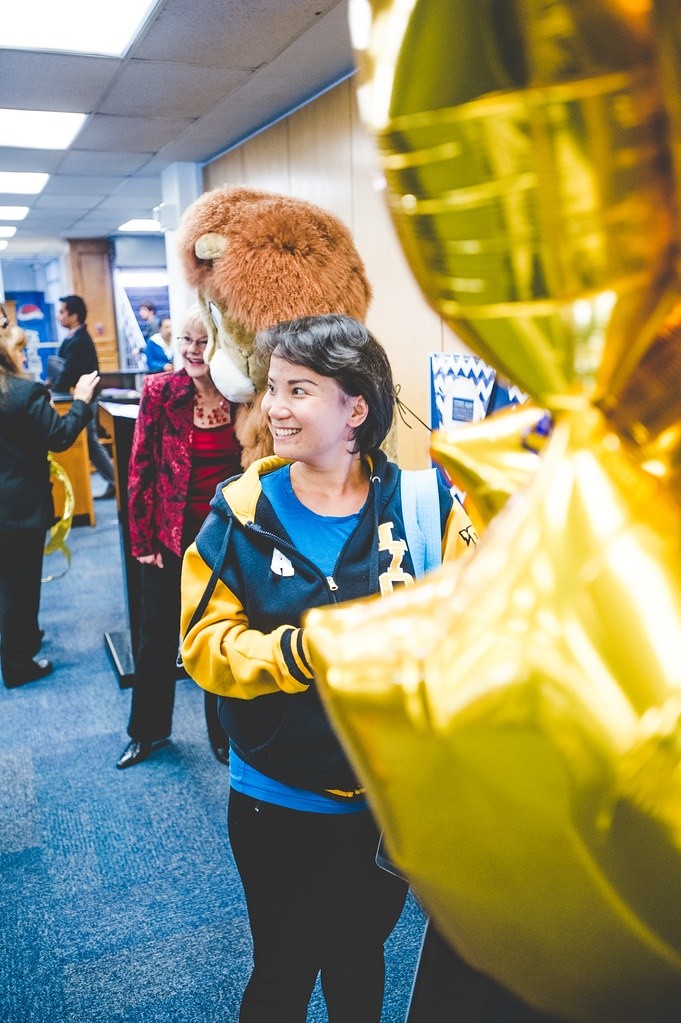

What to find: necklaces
left=193, top=391, right=230, bottom=425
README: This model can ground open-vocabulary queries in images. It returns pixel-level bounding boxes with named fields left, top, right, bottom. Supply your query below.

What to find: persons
left=45, top=295, right=116, bottom=499
left=182, top=314, right=480, bottom=1023
left=117, top=313, right=230, bottom=768
left=133, top=301, right=161, bottom=356
left=145, top=316, right=175, bottom=374
left=0, top=324, right=99, bottom=687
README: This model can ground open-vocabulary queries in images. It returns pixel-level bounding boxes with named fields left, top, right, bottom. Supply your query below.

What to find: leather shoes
left=115, top=740, right=168, bottom=769
left=209, top=735, right=230, bottom=766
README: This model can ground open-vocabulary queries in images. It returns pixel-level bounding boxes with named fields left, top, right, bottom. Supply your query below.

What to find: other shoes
left=40, top=629, right=44, bottom=641
left=5, top=658, right=51, bottom=689
left=94, top=485, right=115, bottom=500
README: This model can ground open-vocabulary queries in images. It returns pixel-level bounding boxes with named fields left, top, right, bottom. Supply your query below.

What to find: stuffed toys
left=179, top=186, right=375, bottom=468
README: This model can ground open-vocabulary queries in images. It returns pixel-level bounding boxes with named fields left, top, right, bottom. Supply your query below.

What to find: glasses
left=177, top=336, right=207, bottom=351
